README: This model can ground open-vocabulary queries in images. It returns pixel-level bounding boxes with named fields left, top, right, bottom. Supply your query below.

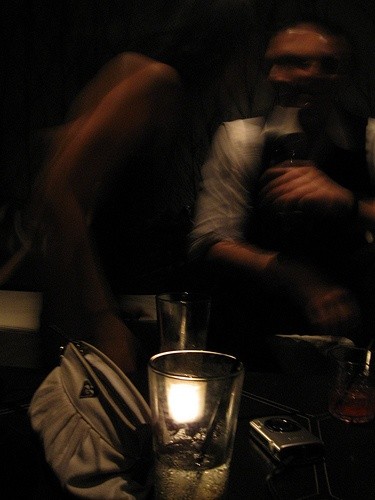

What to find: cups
left=148, top=350, right=246, bottom=500
left=156, top=293, right=212, bottom=351
left=327, top=345, right=375, bottom=424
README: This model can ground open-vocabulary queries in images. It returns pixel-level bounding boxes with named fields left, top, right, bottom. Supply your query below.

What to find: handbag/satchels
left=27, top=338, right=154, bottom=500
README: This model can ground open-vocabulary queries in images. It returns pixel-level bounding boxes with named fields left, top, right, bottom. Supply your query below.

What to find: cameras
left=249, top=416, right=326, bottom=468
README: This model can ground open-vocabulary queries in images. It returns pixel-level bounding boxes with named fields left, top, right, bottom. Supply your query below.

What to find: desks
left=0, top=372, right=375, bottom=500
